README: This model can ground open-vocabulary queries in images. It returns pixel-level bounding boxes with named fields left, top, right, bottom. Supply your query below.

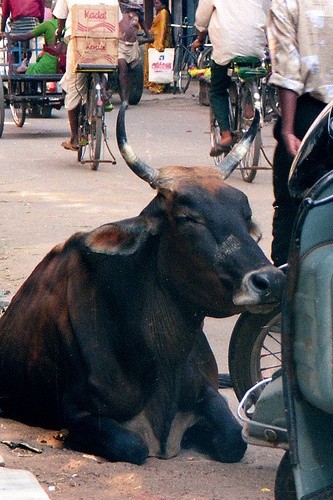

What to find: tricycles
left=0, top=29, right=74, bottom=137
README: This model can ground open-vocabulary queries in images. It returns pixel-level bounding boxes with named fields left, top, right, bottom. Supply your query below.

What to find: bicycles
left=176, top=27, right=215, bottom=92
left=69, top=64, right=122, bottom=170
left=196, top=42, right=278, bottom=182
left=259, top=47, right=284, bottom=120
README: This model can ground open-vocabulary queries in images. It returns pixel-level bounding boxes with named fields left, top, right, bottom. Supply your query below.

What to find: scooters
left=228, top=100, right=333, bottom=499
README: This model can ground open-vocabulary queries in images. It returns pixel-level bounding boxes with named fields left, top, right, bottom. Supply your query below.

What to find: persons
left=52, top=0, right=123, bottom=150
left=102, top=0, right=153, bottom=109
left=191, top=0, right=269, bottom=152
left=2, top=8, right=66, bottom=74
left=265, top=0, right=333, bottom=278
left=0, top=0, right=45, bottom=68
left=144, top=0, right=171, bottom=93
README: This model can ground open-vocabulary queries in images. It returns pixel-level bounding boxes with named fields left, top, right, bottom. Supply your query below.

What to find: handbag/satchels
left=148, top=48, right=175, bottom=84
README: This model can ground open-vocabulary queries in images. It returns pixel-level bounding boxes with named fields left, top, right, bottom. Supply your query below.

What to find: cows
left=1, top=100, right=289, bottom=465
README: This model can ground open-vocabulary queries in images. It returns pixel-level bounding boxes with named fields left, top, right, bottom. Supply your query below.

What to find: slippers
left=242, top=117, right=254, bottom=126
left=209, top=142, right=233, bottom=157
left=62, top=141, right=79, bottom=151
left=101, top=96, right=113, bottom=111
